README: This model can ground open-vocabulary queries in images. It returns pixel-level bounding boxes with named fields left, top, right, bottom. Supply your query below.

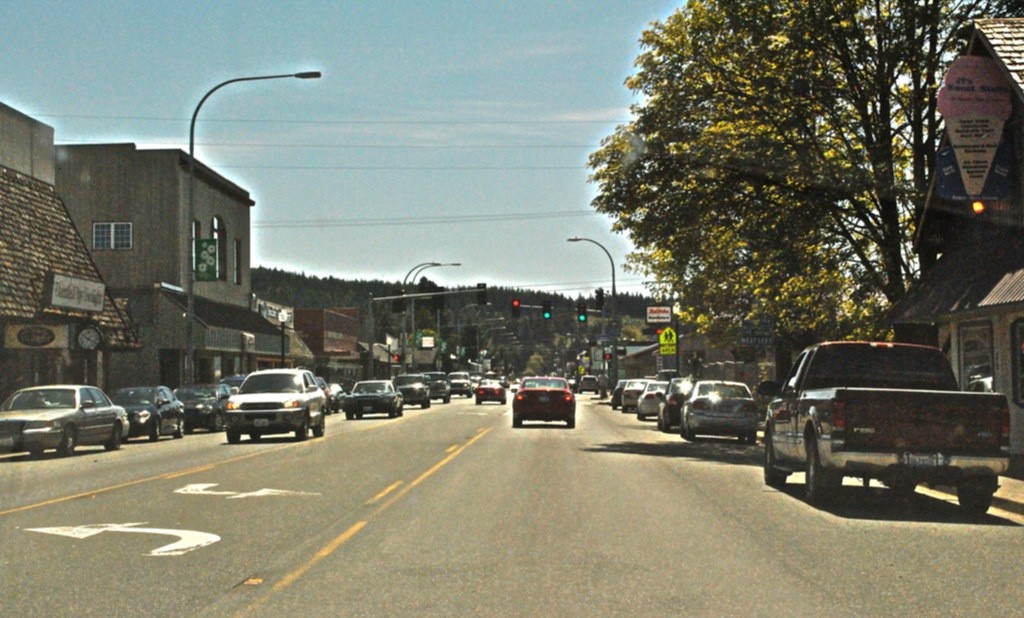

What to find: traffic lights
left=595, top=288, right=604, bottom=309
left=578, top=303, right=587, bottom=323
left=511, top=299, right=520, bottom=317
left=542, top=301, right=551, bottom=319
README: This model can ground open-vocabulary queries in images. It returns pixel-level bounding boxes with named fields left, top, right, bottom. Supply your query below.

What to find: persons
left=574, top=372, right=580, bottom=392
left=598, top=372, right=610, bottom=398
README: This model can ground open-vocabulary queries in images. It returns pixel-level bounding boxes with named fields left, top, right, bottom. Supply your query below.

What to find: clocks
left=75, top=323, right=104, bottom=356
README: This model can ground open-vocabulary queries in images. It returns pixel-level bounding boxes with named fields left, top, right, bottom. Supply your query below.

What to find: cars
left=621, top=378, right=653, bottom=413
left=106, top=385, right=185, bottom=442
left=0, top=384, right=131, bottom=458
left=475, top=379, right=506, bottom=405
left=656, top=377, right=699, bottom=433
left=312, top=377, right=349, bottom=414
left=470, top=373, right=510, bottom=391
left=578, top=375, right=599, bottom=394
left=511, top=377, right=577, bottom=428
left=568, top=379, right=575, bottom=388
left=610, top=379, right=630, bottom=410
left=171, top=382, right=233, bottom=432
left=635, top=381, right=671, bottom=419
left=679, top=379, right=758, bottom=444
left=343, top=379, right=404, bottom=420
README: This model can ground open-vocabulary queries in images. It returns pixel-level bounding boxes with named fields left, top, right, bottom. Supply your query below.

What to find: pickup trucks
left=757, top=340, right=1012, bottom=514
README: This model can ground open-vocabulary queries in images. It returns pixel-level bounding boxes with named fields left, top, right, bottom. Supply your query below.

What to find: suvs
left=395, top=374, right=432, bottom=409
left=423, top=372, right=452, bottom=403
left=447, top=371, right=474, bottom=399
left=223, top=365, right=327, bottom=444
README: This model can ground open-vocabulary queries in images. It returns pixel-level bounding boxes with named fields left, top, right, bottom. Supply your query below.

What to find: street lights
left=411, top=262, right=462, bottom=373
left=567, top=237, right=618, bottom=391
left=182, top=70, right=322, bottom=388
left=476, top=316, right=524, bottom=377
left=400, top=263, right=440, bottom=373
left=458, top=303, right=492, bottom=372
